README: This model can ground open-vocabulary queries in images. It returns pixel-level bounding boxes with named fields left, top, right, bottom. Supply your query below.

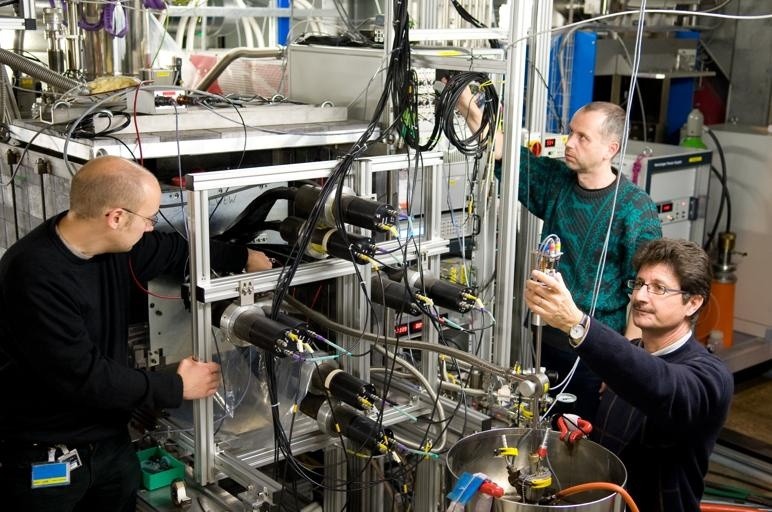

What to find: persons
left=1, top=155, right=272, bottom=512
left=443, top=76, right=662, bottom=430
left=524, top=238, right=733, bottom=512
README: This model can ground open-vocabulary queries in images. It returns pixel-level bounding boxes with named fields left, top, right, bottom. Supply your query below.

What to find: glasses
left=627, top=279, right=689, bottom=295
left=105, top=208, right=159, bottom=226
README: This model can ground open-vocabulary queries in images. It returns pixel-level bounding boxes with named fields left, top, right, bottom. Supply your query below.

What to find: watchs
left=567, top=313, right=588, bottom=341
